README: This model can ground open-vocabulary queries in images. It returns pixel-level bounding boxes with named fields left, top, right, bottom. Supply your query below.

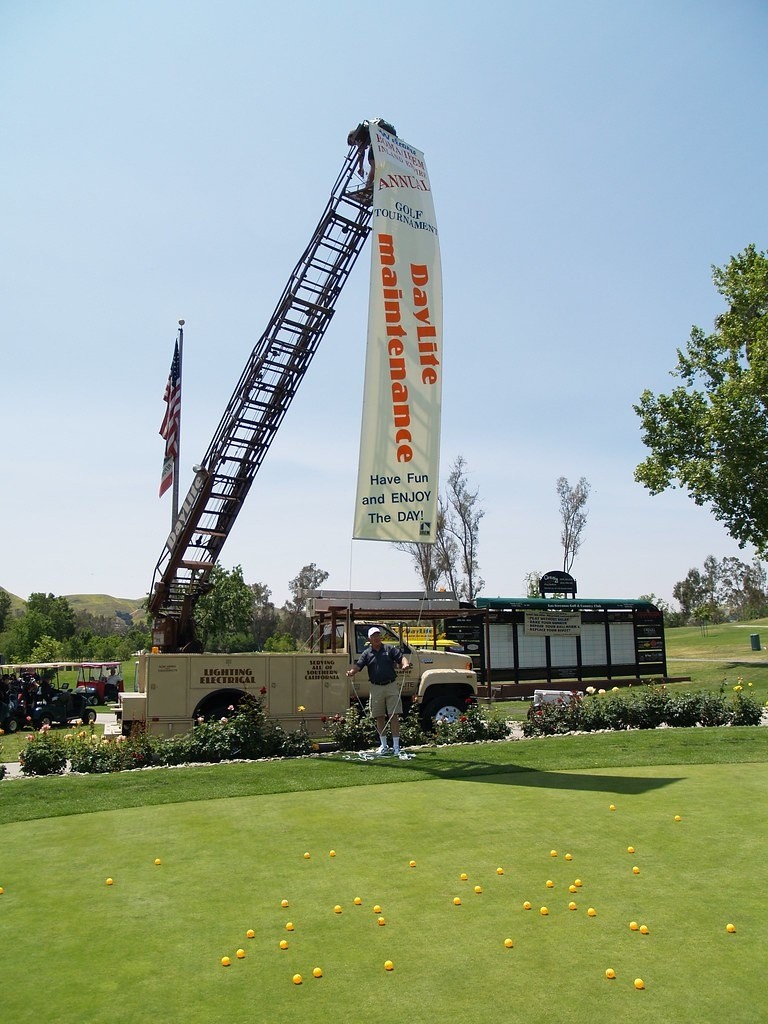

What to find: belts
left=371, top=680, right=396, bottom=686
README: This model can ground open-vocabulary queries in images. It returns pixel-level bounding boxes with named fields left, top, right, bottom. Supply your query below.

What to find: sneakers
left=377, top=744, right=390, bottom=755
left=393, top=745, right=400, bottom=757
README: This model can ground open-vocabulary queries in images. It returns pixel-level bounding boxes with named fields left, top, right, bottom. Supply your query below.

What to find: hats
left=369, top=628, right=381, bottom=639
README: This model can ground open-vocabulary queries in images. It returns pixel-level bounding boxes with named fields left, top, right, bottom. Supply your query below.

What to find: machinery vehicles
left=108, top=117, right=479, bottom=743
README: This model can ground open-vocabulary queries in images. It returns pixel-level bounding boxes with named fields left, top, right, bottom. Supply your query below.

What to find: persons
left=346, top=626, right=410, bottom=756
left=0, top=668, right=123, bottom=716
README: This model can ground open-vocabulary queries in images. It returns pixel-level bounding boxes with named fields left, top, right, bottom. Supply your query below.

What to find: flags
left=159, top=339, right=183, bottom=498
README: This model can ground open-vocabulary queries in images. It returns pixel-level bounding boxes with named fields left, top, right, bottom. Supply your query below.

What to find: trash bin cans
left=750, top=634, right=760, bottom=651
